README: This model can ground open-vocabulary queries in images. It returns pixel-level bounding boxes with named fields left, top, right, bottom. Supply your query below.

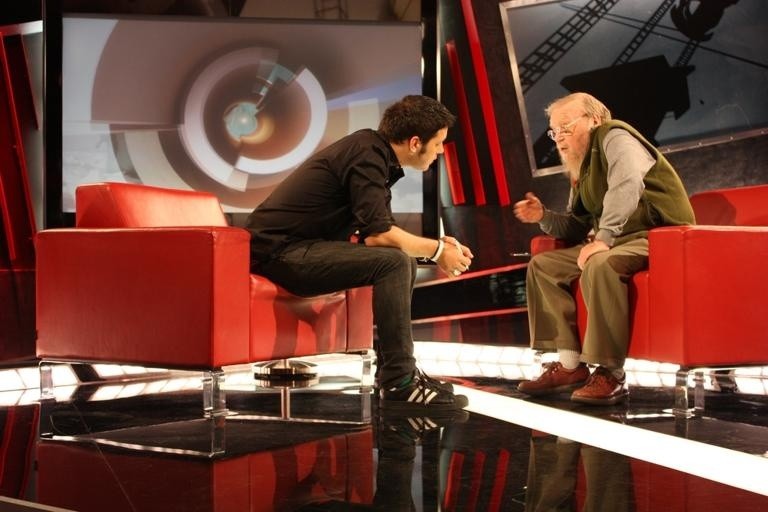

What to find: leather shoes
left=517, top=359, right=590, bottom=397
left=571, top=365, right=631, bottom=405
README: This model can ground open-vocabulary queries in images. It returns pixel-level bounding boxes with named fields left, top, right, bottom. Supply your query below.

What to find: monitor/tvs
left=44, top=12, right=439, bottom=270
left=498, top=0, right=766, bottom=178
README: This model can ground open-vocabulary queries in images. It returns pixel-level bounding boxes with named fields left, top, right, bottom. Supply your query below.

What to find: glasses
left=547, top=116, right=582, bottom=140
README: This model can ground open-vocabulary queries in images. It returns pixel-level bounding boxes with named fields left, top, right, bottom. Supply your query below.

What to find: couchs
left=531, top=186, right=768, bottom=420
left=33, top=183, right=374, bottom=461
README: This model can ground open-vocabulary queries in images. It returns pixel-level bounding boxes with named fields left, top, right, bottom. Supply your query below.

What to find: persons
left=513, top=92, right=696, bottom=406
left=243, top=96, right=473, bottom=414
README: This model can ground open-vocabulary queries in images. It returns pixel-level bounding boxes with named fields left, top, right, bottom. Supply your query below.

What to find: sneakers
left=379, top=372, right=469, bottom=415
left=419, top=371, right=454, bottom=393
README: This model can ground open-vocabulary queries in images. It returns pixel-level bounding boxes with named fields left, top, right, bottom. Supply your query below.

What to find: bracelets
left=429, top=240, right=444, bottom=263
